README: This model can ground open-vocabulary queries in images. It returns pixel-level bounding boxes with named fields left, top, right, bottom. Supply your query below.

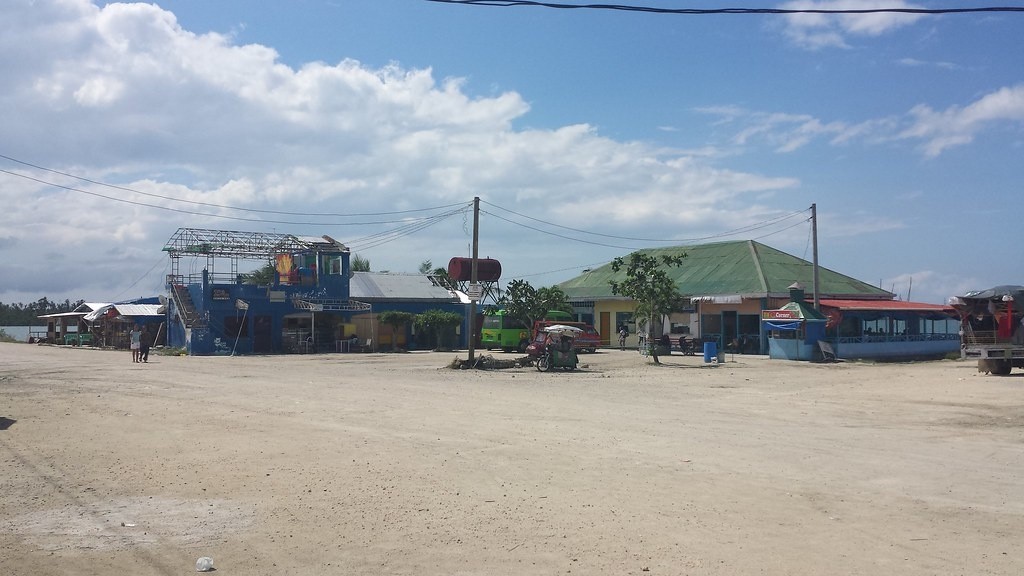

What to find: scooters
left=524, top=330, right=574, bottom=361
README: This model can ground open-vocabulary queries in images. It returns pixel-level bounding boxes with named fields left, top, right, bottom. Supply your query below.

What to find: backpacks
left=622, top=330, right=629, bottom=338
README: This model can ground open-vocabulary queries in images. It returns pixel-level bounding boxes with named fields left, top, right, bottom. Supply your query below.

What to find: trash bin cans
left=704, top=342, right=718, bottom=363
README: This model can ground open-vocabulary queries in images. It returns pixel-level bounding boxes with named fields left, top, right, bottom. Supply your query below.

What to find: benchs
left=64, top=334, right=78, bottom=345
left=79, top=334, right=93, bottom=347
left=653, top=338, right=692, bottom=351
left=350, top=338, right=373, bottom=353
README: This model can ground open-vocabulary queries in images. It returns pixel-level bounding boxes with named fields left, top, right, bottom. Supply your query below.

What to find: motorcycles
left=538, top=345, right=577, bottom=371
left=678, top=335, right=694, bottom=355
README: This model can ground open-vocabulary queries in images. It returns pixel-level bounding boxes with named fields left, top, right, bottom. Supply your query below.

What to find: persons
left=139, top=325, right=151, bottom=363
left=867, top=327, right=872, bottom=334
left=879, top=328, right=884, bottom=333
left=617, top=327, right=626, bottom=351
left=634, top=329, right=646, bottom=346
left=129, top=324, right=142, bottom=363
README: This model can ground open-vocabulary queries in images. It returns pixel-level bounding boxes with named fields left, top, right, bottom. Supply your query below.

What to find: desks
left=335, top=339, right=351, bottom=352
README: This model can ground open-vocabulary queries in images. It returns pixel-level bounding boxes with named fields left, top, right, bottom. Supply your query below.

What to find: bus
left=481, top=310, right=574, bottom=353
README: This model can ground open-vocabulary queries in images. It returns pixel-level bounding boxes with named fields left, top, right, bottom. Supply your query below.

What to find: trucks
left=532, top=321, right=602, bottom=354
